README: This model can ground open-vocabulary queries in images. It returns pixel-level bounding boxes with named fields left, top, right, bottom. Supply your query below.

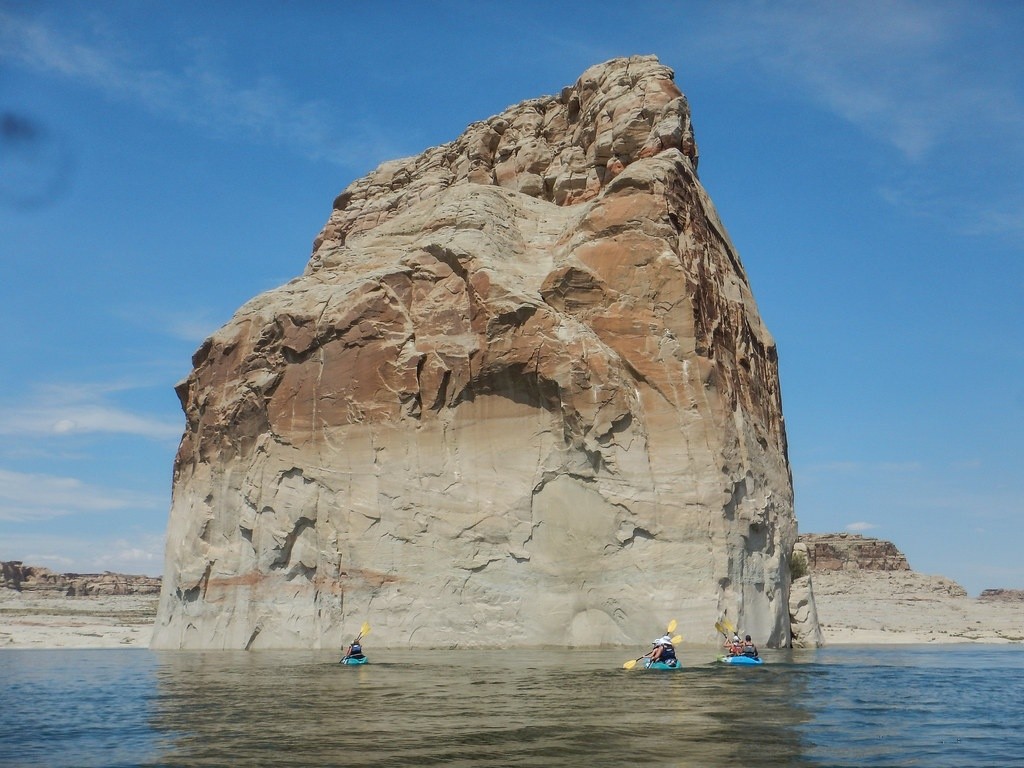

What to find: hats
left=657, top=636, right=672, bottom=646
left=733, top=636, right=739, bottom=641
left=652, top=639, right=660, bottom=644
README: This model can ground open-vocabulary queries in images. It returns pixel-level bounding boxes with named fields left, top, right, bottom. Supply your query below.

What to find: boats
left=717, top=653, right=763, bottom=665
left=340, top=656, right=369, bottom=664
left=643, top=657, right=682, bottom=669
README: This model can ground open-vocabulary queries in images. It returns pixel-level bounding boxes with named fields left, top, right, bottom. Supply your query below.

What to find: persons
left=346, top=639, right=365, bottom=659
left=734, top=635, right=759, bottom=661
left=652, top=636, right=677, bottom=665
left=643, top=639, right=660, bottom=663
left=724, top=636, right=744, bottom=657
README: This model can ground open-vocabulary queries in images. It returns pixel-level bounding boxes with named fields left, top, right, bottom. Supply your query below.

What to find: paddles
left=715, top=621, right=732, bottom=644
left=623, top=634, right=683, bottom=669
left=647, top=619, right=677, bottom=669
left=723, top=618, right=741, bottom=640
left=339, top=622, right=372, bottom=664
